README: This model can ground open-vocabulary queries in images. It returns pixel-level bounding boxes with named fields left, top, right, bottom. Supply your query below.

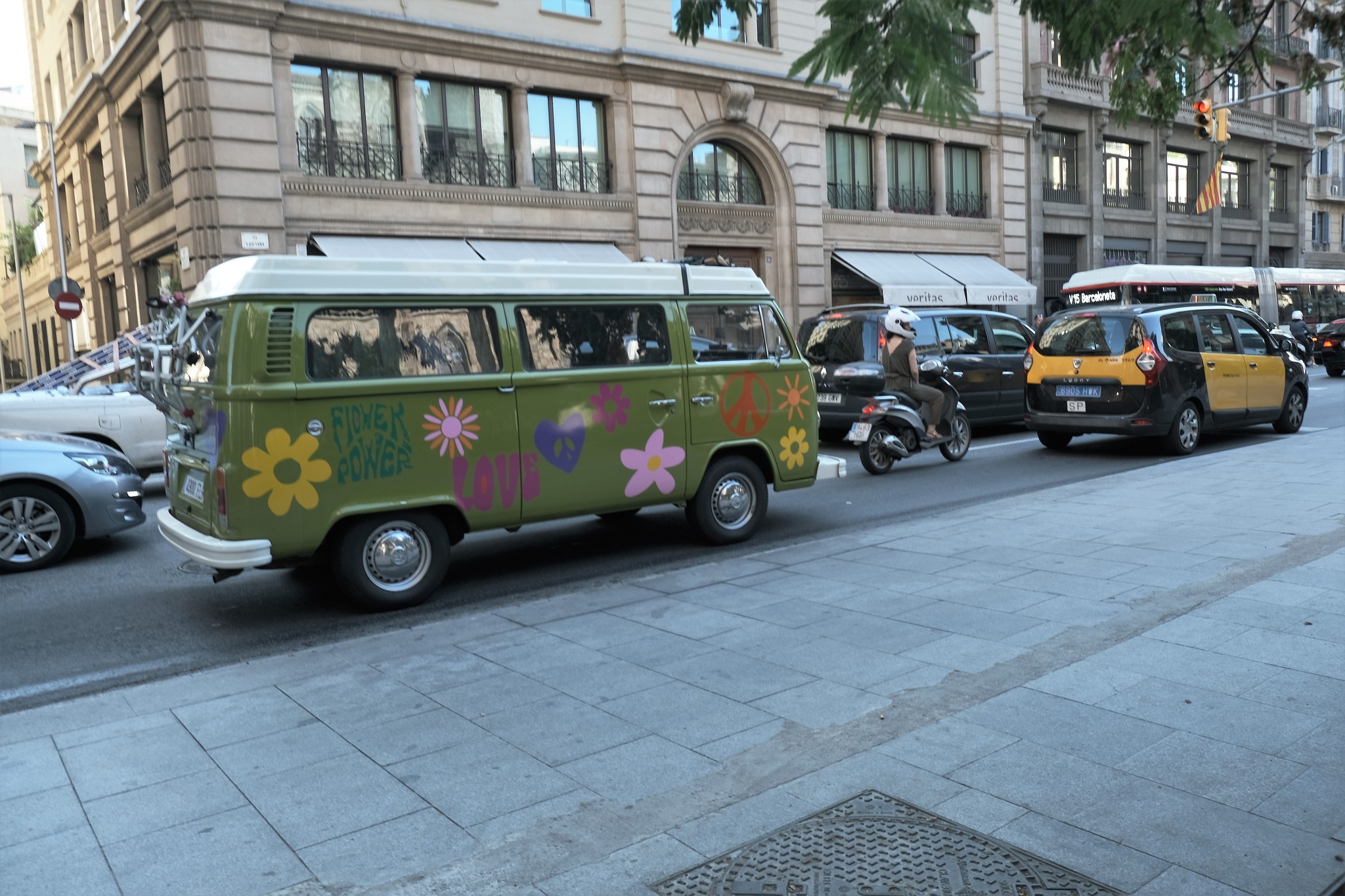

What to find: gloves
left=1312, top=332, right=1316, bottom=335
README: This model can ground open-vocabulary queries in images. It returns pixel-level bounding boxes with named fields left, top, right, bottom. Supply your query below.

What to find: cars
left=1320, top=318, right=1345, bottom=377
left=0, top=429, right=146, bottom=571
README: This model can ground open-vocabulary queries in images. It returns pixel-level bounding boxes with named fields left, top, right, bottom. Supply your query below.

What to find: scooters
left=1295, top=324, right=1320, bottom=365
left=849, top=341, right=971, bottom=475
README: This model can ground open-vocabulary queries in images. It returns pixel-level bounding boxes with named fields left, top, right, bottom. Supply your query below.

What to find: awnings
left=831, top=250, right=1037, bottom=305
left=308, top=234, right=628, bottom=263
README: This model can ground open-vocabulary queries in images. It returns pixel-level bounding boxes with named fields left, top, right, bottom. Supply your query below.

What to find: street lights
left=13, top=120, right=76, bottom=390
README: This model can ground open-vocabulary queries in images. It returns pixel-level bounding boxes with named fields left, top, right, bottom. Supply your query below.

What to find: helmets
left=1292, top=310, right=1303, bottom=320
left=885, top=307, right=920, bottom=340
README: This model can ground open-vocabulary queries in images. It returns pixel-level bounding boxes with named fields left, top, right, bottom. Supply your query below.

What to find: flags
left=1196, top=154, right=1223, bottom=214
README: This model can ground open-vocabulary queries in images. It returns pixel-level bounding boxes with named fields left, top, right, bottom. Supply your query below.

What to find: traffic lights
left=1215, top=103, right=1232, bottom=142
left=1192, top=98, right=1212, bottom=140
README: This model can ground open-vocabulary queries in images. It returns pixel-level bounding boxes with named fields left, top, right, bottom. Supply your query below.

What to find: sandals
left=926, top=431, right=943, bottom=440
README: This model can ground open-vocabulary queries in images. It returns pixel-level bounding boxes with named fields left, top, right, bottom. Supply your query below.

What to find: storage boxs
left=832, top=360, right=886, bottom=396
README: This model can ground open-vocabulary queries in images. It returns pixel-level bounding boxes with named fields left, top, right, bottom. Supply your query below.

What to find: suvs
left=796, top=304, right=1037, bottom=440
left=1023, top=302, right=1310, bottom=456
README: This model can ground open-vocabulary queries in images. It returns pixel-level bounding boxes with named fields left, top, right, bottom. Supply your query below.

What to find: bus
left=1062, top=264, right=1345, bottom=358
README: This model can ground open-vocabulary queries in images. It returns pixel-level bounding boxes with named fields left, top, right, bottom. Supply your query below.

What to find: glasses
left=1034, top=314, right=1043, bottom=319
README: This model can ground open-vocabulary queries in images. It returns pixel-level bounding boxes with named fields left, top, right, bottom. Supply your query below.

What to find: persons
left=1031, top=314, right=1043, bottom=330
left=1289, top=310, right=1320, bottom=367
left=882, top=307, right=944, bottom=439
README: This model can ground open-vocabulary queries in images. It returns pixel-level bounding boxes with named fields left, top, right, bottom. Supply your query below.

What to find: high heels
left=1312, top=362, right=1318, bottom=368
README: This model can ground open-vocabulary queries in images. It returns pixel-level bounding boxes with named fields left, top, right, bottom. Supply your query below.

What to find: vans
left=126, top=255, right=847, bottom=610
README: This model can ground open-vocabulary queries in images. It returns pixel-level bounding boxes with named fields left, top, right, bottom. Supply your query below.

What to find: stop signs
left=54, top=293, right=82, bottom=321
